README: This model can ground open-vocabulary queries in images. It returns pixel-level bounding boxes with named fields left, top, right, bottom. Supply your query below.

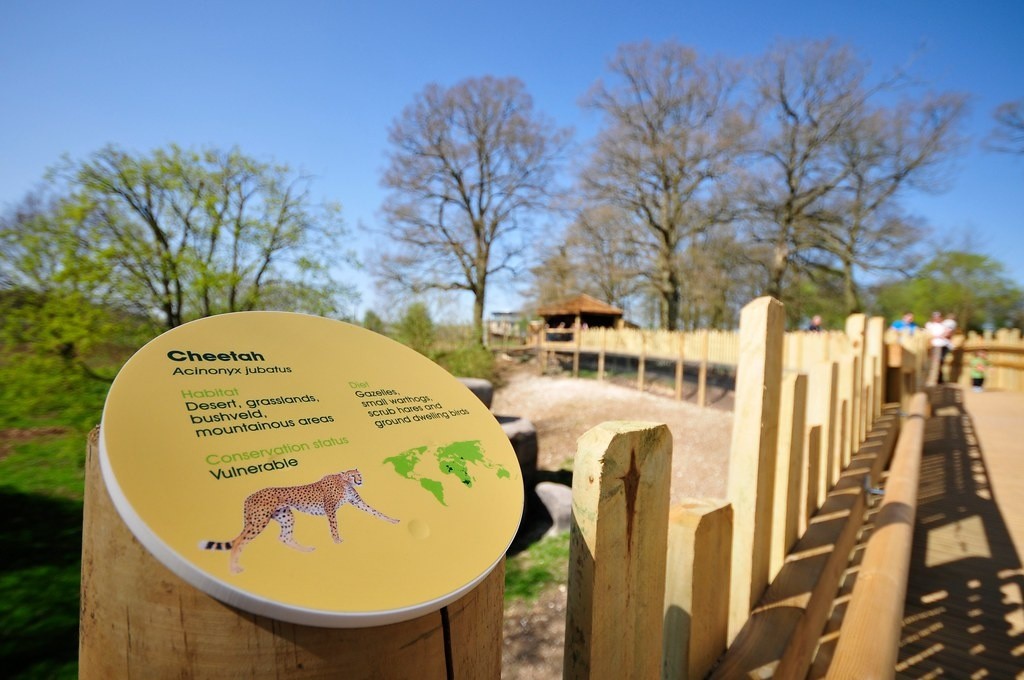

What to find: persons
left=545, top=323, right=588, bottom=341
left=888, top=310, right=920, bottom=336
left=969, top=349, right=991, bottom=392
left=924, top=310, right=957, bottom=384
left=803, top=316, right=826, bottom=334
